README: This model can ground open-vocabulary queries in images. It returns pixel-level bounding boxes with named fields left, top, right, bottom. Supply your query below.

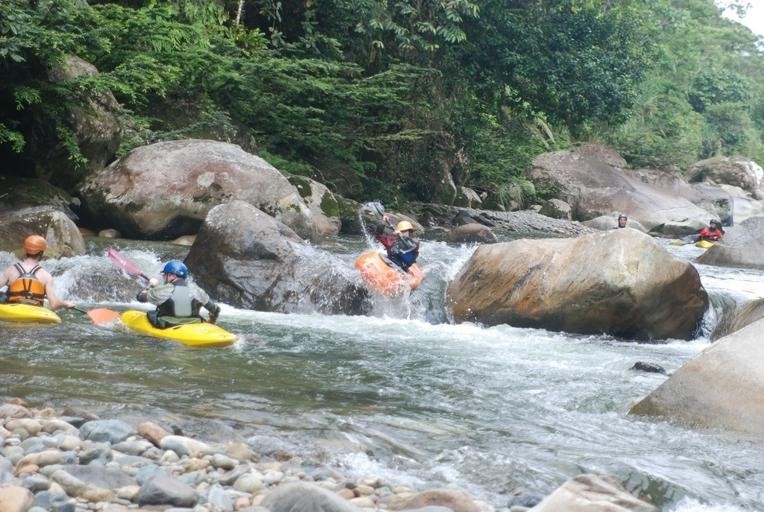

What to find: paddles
left=107, top=249, right=149, bottom=281
left=373, top=203, right=415, bottom=251
left=71, top=308, right=121, bottom=325
left=670, top=237, right=718, bottom=246
left=646, top=223, right=665, bottom=235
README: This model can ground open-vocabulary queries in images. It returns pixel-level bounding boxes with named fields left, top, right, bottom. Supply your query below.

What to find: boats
left=0, top=303, right=62, bottom=324
left=121, top=309, right=237, bottom=348
left=669, top=240, right=719, bottom=248
left=355, top=250, right=425, bottom=301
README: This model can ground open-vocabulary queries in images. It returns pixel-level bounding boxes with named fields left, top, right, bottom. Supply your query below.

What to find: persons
left=615, top=211, right=631, bottom=228
left=376, top=215, right=419, bottom=271
left=134, top=260, right=220, bottom=326
left=0, top=233, right=76, bottom=314
left=690, top=218, right=724, bottom=244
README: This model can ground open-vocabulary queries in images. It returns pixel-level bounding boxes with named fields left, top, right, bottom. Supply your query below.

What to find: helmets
left=160, top=260, right=188, bottom=279
left=397, top=221, right=414, bottom=233
left=618, top=214, right=627, bottom=221
left=710, top=219, right=718, bottom=224
left=23, top=235, right=47, bottom=256
